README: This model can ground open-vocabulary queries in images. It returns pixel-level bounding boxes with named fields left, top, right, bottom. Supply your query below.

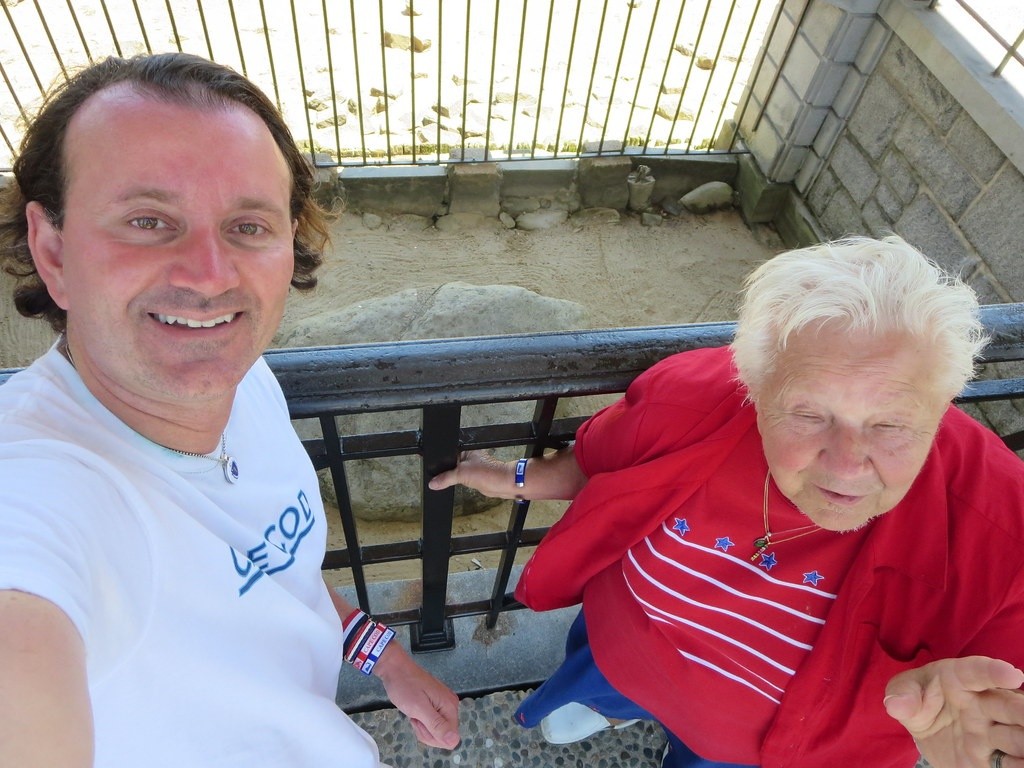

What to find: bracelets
left=342, top=608, right=396, bottom=676
left=515, top=458, right=528, bottom=504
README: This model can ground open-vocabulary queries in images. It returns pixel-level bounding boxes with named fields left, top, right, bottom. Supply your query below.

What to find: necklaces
left=65, top=342, right=240, bottom=484
left=750, top=467, right=822, bottom=562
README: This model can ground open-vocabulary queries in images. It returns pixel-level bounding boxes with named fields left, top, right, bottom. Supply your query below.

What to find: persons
left=1, top=56, right=458, bottom=768
left=428, top=230, right=1024, bottom=768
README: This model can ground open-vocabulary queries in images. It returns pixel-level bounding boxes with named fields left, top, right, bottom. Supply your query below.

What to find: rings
left=989, top=749, right=1007, bottom=768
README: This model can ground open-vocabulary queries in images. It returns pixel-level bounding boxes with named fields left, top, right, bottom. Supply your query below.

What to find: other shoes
left=661, top=741, right=670, bottom=768
left=541, top=701, right=642, bottom=744
